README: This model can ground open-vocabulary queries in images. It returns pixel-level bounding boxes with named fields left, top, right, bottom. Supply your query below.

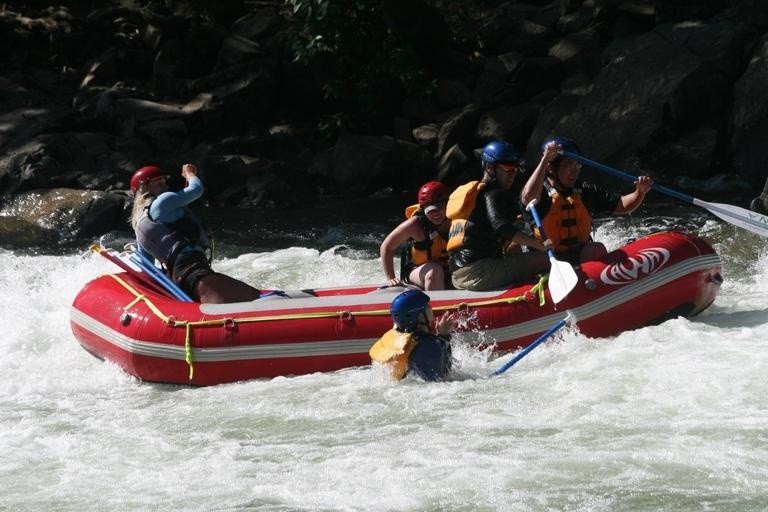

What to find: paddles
left=525, top=198, right=578, bottom=305
left=556, top=146, right=768, bottom=239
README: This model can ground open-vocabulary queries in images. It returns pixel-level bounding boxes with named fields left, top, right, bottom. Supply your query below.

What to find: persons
left=442, top=143, right=558, bottom=292
left=380, top=181, right=453, bottom=291
left=130, top=165, right=261, bottom=304
left=520, top=140, right=656, bottom=263
left=368, top=287, right=457, bottom=382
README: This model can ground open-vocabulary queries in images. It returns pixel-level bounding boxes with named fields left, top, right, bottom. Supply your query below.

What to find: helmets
left=481, top=139, right=520, bottom=164
left=131, top=167, right=164, bottom=191
left=539, top=136, right=578, bottom=156
left=418, top=180, right=450, bottom=203
left=391, top=289, right=431, bottom=317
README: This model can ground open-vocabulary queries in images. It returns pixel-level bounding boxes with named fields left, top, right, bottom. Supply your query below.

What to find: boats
left=66, top=219, right=730, bottom=391
